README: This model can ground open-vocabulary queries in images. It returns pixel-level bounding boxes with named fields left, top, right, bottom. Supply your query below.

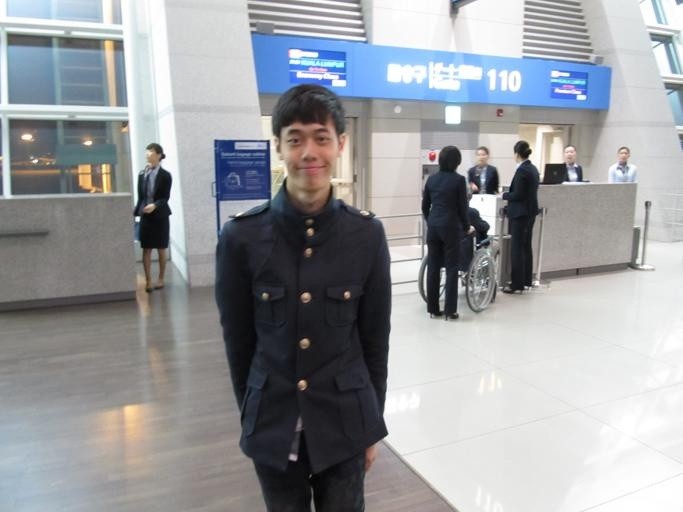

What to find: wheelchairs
left=418, top=218, right=499, bottom=313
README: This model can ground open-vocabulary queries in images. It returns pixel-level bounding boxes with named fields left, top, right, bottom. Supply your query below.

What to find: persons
left=562, top=144, right=583, bottom=182
left=499, top=140, right=540, bottom=294
left=215, top=83, right=392, bottom=512
left=468, top=146, right=499, bottom=194
left=422, top=140, right=476, bottom=320
left=467, top=193, right=500, bottom=303
left=609, top=147, right=638, bottom=183
left=134, top=142, right=172, bottom=292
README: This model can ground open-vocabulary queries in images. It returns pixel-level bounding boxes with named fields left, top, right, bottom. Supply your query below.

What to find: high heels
left=445, top=311, right=459, bottom=321
left=429, top=311, right=444, bottom=318
left=501, top=288, right=524, bottom=294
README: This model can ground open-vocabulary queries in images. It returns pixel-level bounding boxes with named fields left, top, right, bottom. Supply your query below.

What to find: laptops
left=539, top=164, right=566, bottom=185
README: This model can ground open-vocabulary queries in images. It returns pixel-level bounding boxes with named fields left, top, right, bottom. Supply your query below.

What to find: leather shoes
left=146, top=283, right=153, bottom=292
